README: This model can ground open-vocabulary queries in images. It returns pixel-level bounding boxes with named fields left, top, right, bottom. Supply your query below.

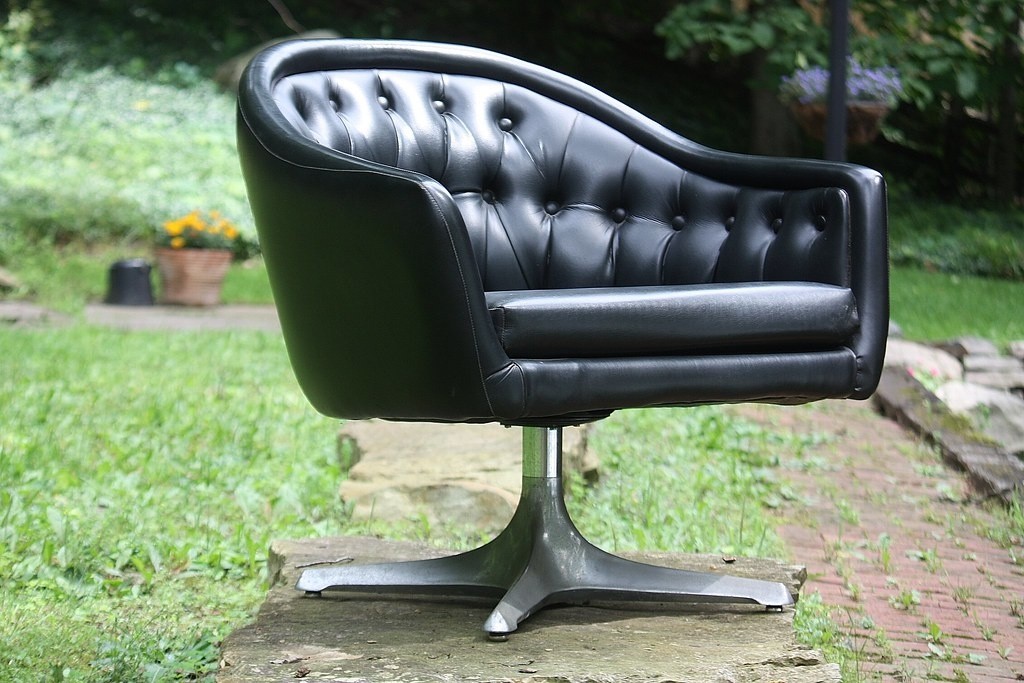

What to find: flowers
left=154, top=208, right=263, bottom=268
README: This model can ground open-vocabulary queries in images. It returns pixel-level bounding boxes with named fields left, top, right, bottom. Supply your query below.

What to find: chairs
left=235, top=38, right=890, bottom=643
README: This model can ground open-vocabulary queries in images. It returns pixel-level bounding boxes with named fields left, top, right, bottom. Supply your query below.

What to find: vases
left=155, top=245, right=233, bottom=306
left=104, top=257, right=156, bottom=307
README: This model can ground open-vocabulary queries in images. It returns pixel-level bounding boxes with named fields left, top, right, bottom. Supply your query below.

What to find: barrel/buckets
left=102, top=259, right=158, bottom=305
